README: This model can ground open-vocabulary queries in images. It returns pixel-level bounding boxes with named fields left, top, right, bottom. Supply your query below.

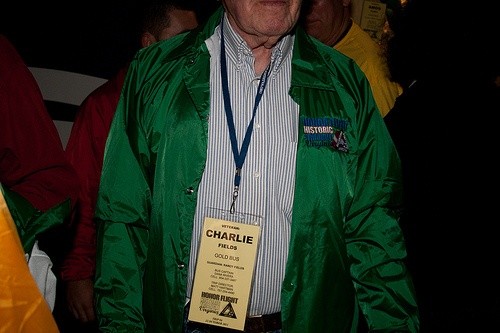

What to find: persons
left=0, top=5, right=82, bottom=333
left=60, top=0, right=407, bottom=333
